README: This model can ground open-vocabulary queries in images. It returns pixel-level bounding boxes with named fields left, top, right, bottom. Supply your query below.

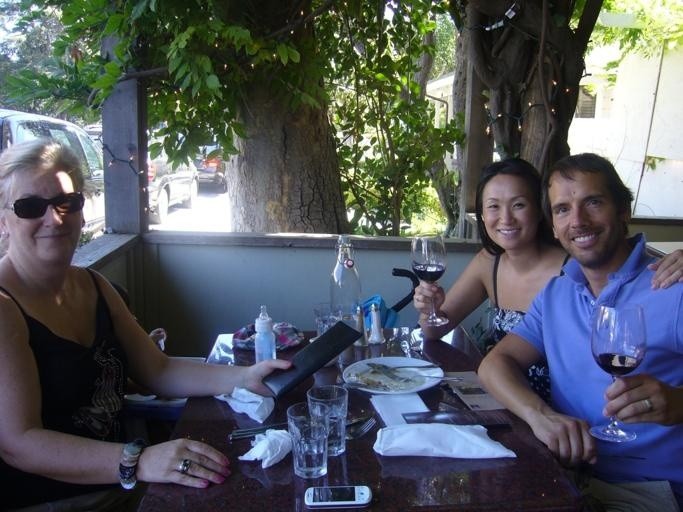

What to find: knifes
left=227, top=415, right=367, bottom=440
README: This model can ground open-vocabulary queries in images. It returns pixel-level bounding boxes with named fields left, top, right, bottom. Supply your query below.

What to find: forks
left=381, top=370, right=464, bottom=383
left=326, top=417, right=376, bottom=444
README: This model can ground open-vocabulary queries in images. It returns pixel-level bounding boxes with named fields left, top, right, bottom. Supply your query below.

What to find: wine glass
left=410, top=235, right=449, bottom=327
left=588, top=301, right=647, bottom=443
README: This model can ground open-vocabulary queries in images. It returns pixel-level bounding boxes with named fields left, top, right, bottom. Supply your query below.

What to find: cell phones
left=305, top=485, right=372, bottom=507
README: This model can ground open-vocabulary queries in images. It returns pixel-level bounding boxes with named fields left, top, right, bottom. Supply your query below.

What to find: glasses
left=0, top=188, right=86, bottom=222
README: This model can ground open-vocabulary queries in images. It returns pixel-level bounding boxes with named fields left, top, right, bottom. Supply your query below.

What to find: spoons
left=365, top=360, right=441, bottom=373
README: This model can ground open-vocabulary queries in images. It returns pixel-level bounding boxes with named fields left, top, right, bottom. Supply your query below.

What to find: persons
left=1, top=135, right=294, bottom=510
left=411, top=155, right=681, bottom=401
left=476, top=153, right=683, bottom=512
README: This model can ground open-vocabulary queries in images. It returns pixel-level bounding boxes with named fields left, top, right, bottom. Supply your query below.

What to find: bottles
left=328, top=235, right=361, bottom=325
left=367, top=302, right=386, bottom=344
left=254, top=304, right=276, bottom=363
left=352, top=306, right=368, bottom=347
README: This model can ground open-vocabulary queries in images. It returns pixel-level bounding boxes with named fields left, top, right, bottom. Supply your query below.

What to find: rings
left=643, top=398, right=652, bottom=412
left=178, top=457, right=190, bottom=474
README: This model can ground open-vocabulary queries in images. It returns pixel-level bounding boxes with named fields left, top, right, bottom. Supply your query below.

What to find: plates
left=342, top=356, right=443, bottom=394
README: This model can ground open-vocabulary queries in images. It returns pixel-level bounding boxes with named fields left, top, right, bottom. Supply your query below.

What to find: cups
left=287, top=403, right=329, bottom=480
left=313, top=303, right=343, bottom=337
left=307, top=383, right=348, bottom=456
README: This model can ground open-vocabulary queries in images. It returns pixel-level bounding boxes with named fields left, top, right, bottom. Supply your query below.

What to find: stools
left=123, top=356, right=208, bottom=441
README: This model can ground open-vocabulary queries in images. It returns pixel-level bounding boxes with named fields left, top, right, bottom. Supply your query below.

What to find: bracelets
left=115, top=435, right=144, bottom=491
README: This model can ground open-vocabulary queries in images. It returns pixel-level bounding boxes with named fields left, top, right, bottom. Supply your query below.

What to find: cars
left=0, top=104, right=235, bottom=257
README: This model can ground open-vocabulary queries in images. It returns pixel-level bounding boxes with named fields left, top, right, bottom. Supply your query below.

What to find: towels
left=367, top=417, right=519, bottom=462
left=212, top=379, right=276, bottom=426
